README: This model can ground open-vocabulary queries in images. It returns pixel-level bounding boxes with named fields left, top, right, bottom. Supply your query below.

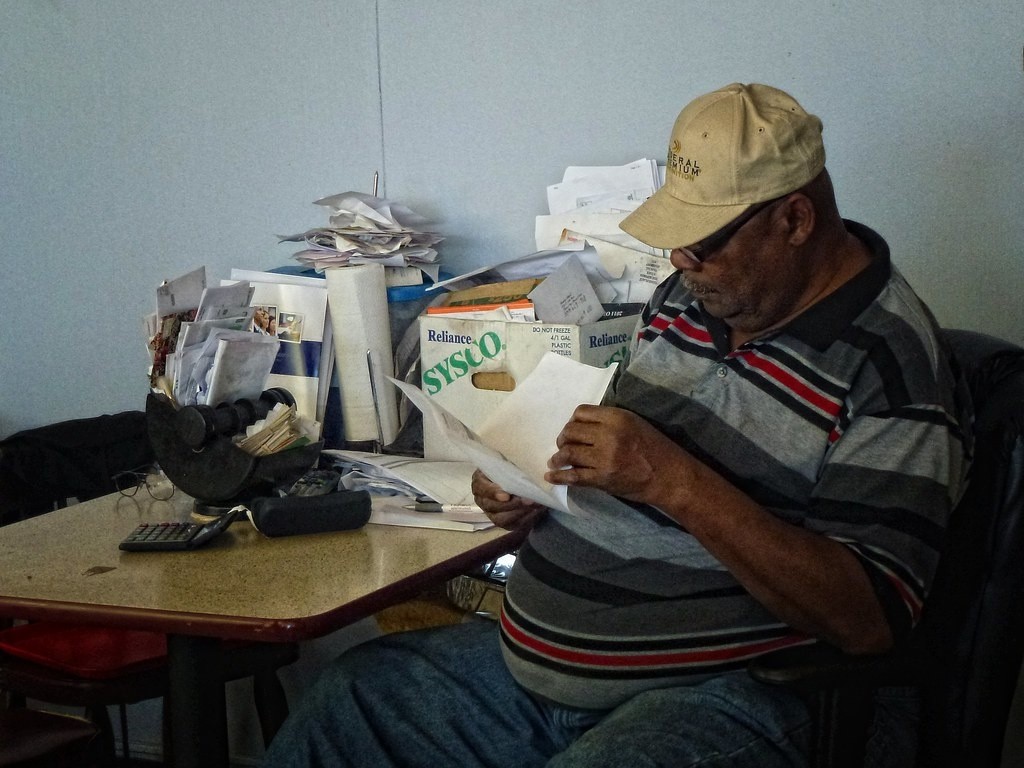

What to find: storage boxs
left=419, top=302, right=647, bottom=460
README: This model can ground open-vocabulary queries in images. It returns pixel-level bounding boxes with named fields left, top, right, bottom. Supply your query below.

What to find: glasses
left=679, top=200, right=773, bottom=262
left=111, top=465, right=174, bottom=501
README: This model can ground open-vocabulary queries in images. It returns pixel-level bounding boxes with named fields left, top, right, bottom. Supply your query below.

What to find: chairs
left=0, top=409, right=299, bottom=768
left=852, top=327, right=1024, bottom=768
left=0, top=705, right=107, bottom=768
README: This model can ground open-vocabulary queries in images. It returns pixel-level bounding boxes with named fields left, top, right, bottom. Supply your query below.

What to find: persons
left=253, top=307, right=275, bottom=336
left=257, top=84, right=975, bottom=767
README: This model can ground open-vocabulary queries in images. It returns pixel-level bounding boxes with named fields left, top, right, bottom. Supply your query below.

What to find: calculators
left=118, top=511, right=239, bottom=550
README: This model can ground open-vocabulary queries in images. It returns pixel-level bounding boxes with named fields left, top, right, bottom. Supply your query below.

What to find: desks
left=0, top=473, right=526, bottom=768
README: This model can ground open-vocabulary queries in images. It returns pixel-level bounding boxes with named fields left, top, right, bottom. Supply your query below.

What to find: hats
left=619, top=82, right=825, bottom=250
